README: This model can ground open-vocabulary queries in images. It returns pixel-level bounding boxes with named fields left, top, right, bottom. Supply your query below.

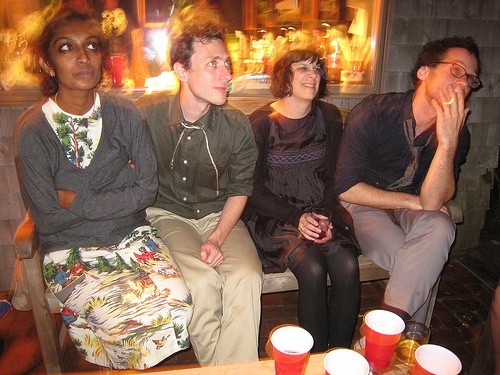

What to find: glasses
left=426, top=62, right=482, bottom=89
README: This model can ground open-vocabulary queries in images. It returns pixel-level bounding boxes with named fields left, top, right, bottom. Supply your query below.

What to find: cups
left=311, top=208, right=332, bottom=240
left=269, top=325, right=315, bottom=375
left=395, top=321, right=430, bottom=365
left=408, top=344, right=463, bottom=375
left=323, top=346, right=371, bottom=375
left=362, top=309, right=406, bottom=375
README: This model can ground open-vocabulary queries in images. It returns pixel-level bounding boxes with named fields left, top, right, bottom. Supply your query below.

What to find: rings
left=300, top=226, right=304, bottom=231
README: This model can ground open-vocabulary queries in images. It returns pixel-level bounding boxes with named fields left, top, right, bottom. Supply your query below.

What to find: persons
left=14, top=8, right=192, bottom=372
left=138, top=22, right=263, bottom=368
left=240, top=48, right=363, bottom=354
left=334, top=33, right=483, bottom=349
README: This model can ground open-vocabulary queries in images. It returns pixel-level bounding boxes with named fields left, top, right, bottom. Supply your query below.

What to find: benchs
left=11, top=93, right=464, bottom=375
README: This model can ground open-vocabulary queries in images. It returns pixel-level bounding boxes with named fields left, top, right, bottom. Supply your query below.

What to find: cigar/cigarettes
left=454, top=86, right=459, bottom=94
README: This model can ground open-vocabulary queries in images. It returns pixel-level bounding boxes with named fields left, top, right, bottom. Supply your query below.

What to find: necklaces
left=53, top=89, right=96, bottom=107
